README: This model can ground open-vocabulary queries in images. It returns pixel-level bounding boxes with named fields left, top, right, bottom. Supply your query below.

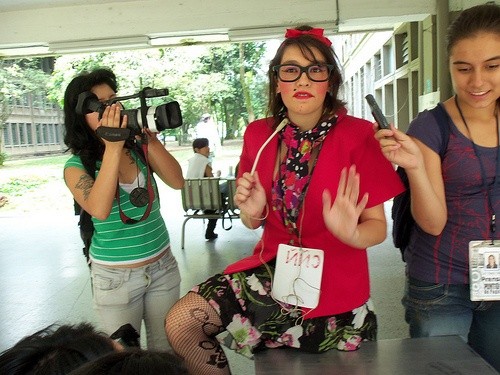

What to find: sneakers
left=206, top=232, right=218, bottom=239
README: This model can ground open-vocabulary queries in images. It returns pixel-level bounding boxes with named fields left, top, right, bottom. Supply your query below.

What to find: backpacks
left=391, top=104, right=451, bottom=260
left=73, top=141, right=160, bottom=271
left=220, top=182, right=233, bottom=230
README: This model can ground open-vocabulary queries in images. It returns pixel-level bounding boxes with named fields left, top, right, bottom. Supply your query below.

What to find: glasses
left=272, top=63, right=335, bottom=82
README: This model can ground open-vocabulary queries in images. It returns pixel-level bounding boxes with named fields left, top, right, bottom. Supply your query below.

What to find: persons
left=374, top=6, right=500, bottom=374
left=190, top=113, right=222, bottom=177
left=63, top=67, right=186, bottom=355
left=0, top=318, right=189, bottom=375
left=163, top=25, right=406, bottom=375
left=185, top=137, right=221, bottom=240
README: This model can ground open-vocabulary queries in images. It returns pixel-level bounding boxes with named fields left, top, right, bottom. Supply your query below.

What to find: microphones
left=141, top=87, right=169, bottom=97
left=249, top=117, right=288, bottom=177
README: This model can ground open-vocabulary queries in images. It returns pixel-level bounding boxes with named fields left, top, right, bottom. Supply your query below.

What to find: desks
left=254, top=332, right=500, bottom=375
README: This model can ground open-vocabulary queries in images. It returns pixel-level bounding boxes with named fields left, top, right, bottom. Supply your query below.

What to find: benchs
left=180, top=175, right=241, bottom=250
